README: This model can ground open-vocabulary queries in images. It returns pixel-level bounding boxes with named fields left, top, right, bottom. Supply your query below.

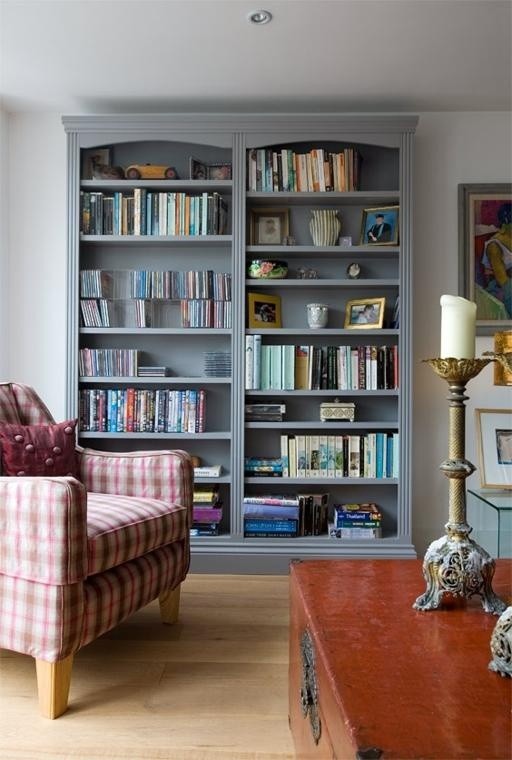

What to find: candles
left=439, top=294, right=478, bottom=359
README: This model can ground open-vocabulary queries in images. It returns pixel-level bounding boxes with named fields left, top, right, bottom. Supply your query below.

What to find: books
left=79, top=390, right=206, bottom=433
left=243, top=333, right=398, bottom=539
left=80, top=267, right=230, bottom=328
left=190, top=491, right=223, bottom=537
left=78, top=190, right=227, bottom=236
left=247, top=147, right=361, bottom=193
left=192, top=457, right=222, bottom=477
left=79, top=348, right=231, bottom=378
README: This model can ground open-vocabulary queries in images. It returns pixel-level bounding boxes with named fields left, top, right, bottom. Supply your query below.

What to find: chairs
left=0, top=380, right=195, bottom=720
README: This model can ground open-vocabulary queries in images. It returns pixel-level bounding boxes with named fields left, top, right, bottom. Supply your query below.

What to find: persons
left=352, top=305, right=374, bottom=322
left=265, top=219, right=277, bottom=235
left=355, top=311, right=367, bottom=323
left=480, top=204, right=511, bottom=319
left=367, top=213, right=392, bottom=243
left=259, top=304, right=275, bottom=322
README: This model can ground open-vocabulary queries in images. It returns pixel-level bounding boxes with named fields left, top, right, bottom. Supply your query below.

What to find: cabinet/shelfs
left=63, top=111, right=421, bottom=576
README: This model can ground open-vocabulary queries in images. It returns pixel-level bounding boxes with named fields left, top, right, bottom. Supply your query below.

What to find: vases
left=307, top=208, right=343, bottom=246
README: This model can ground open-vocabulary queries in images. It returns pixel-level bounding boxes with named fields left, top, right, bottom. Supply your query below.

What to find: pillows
left=0, top=417, right=83, bottom=483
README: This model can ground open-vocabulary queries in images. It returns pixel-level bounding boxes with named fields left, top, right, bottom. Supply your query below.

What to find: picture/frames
left=343, top=297, right=386, bottom=329
left=359, top=205, right=400, bottom=247
left=247, top=292, right=281, bottom=329
left=250, top=205, right=290, bottom=245
left=458, top=182, right=511, bottom=338
left=474, top=408, right=511, bottom=490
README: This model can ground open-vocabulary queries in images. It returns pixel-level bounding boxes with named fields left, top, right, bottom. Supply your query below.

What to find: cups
left=306, top=302, right=329, bottom=328
